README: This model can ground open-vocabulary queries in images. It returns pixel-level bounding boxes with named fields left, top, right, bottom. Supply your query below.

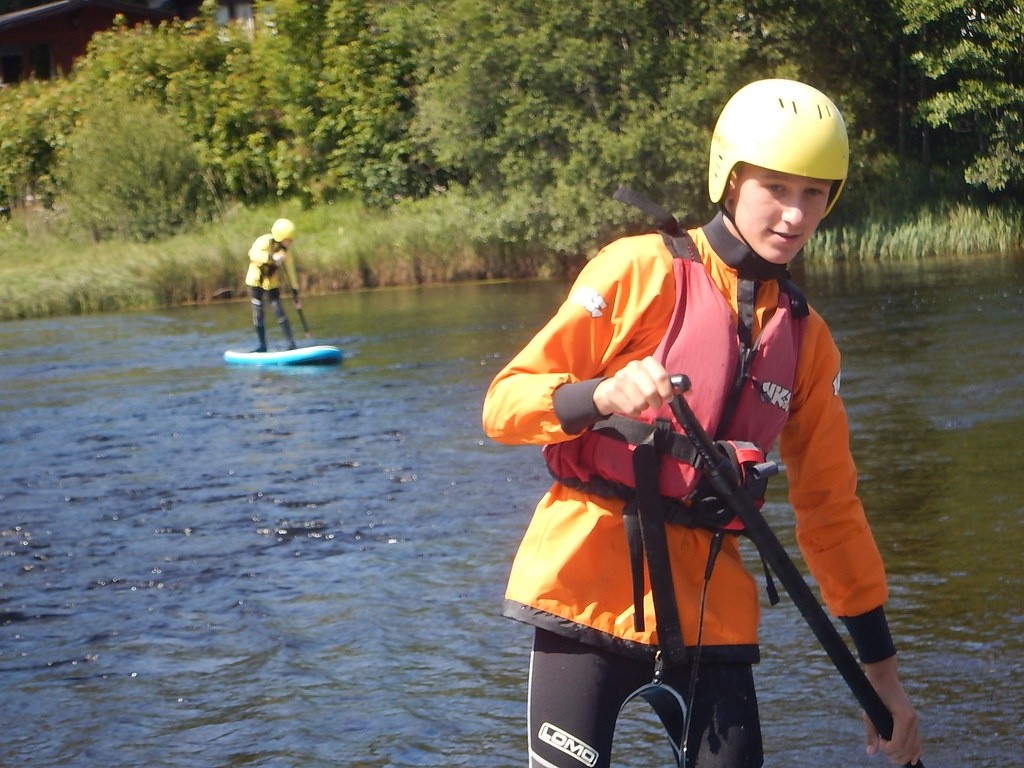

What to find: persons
left=245, top=218, right=302, bottom=352
left=481, top=79, right=923, bottom=768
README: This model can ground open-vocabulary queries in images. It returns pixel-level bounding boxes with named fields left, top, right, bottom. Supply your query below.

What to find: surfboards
left=223, top=345, right=343, bottom=365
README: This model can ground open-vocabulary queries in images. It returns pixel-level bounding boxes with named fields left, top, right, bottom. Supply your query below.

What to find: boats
left=232, top=344, right=343, bottom=369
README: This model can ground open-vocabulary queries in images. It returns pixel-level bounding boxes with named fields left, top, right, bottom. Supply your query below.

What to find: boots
left=280, top=320, right=296, bottom=351
left=250, top=325, right=267, bottom=353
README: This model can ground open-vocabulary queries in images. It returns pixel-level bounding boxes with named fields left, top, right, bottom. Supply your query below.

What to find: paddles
left=276, top=247, right=313, bottom=340
left=663, top=375, right=925, bottom=768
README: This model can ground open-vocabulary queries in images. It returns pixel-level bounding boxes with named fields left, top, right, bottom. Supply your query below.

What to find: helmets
left=272, top=217, right=297, bottom=243
left=708, top=80, right=850, bottom=222
